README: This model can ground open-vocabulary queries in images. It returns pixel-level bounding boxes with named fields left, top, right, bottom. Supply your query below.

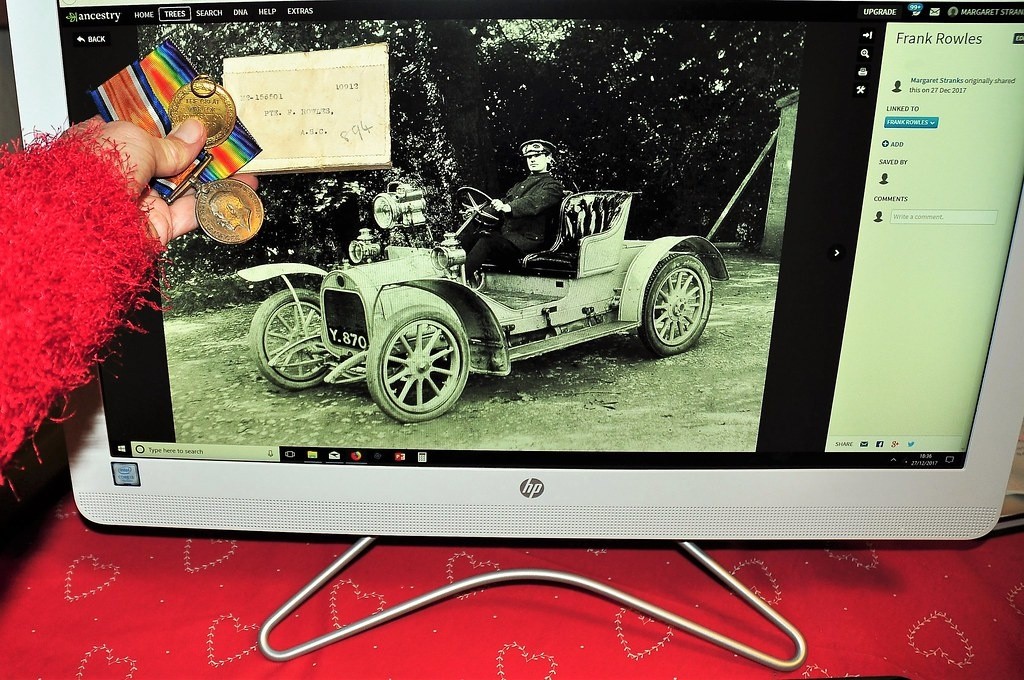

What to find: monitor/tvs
left=1, top=2, right=1023, bottom=544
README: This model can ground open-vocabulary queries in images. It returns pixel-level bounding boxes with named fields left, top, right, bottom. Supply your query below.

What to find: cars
left=238, top=180, right=734, bottom=426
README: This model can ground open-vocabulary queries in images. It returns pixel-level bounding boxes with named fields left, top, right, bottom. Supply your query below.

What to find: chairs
left=455, top=187, right=637, bottom=281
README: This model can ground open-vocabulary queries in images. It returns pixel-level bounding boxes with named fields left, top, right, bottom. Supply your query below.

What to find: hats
left=519, top=139, right=556, bottom=155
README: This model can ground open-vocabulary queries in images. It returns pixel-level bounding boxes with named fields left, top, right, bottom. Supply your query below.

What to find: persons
left=465, top=139, right=564, bottom=289
left=0, top=119, right=259, bottom=472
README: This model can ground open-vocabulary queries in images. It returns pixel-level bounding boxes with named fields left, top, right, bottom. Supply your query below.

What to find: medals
left=168, top=80, right=237, bottom=150
left=195, top=178, right=263, bottom=244
